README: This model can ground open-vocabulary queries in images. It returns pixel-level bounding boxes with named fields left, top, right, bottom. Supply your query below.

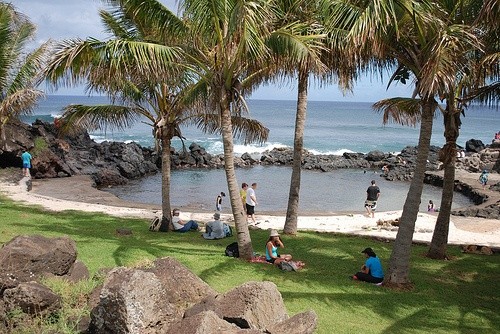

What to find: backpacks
left=149, top=215, right=162, bottom=231
left=160, top=217, right=169, bottom=232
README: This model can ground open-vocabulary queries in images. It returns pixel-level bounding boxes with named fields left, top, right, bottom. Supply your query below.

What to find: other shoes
left=255, top=222, right=261, bottom=225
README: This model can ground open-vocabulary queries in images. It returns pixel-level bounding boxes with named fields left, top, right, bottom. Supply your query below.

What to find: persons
left=264, top=229, right=293, bottom=266
left=245, top=182, right=260, bottom=225
left=479, top=169, right=489, bottom=189
left=240, top=183, right=251, bottom=219
left=172, top=208, right=202, bottom=232
left=383, top=165, right=388, bottom=174
left=428, top=200, right=435, bottom=212
left=216, top=192, right=226, bottom=212
left=352, top=248, right=384, bottom=283
left=22, top=150, right=32, bottom=177
left=364, top=180, right=381, bottom=218
left=205, top=213, right=224, bottom=238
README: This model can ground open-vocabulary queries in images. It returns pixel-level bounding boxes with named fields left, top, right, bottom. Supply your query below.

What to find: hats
left=214, top=213, right=221, bottom=219
left=270, top=229, right=280, bottom=237
